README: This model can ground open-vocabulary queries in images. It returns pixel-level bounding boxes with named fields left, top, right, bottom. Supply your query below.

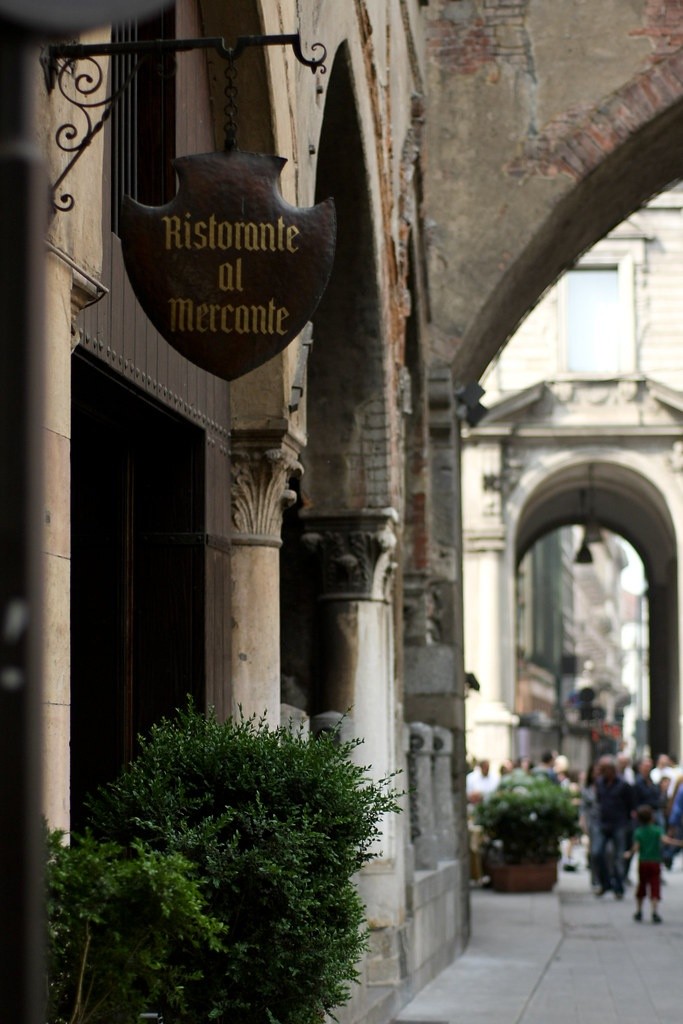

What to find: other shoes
left=635, top=912, right=641, bottom=920
left=597, top=884, right=609, bottom=895
left=652, top=914, right=661, bottom=922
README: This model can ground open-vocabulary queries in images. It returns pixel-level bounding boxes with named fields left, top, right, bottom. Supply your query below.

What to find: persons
left=466, top=749, right=683, bottom=900
left=622, top=804, right=683, bottom=922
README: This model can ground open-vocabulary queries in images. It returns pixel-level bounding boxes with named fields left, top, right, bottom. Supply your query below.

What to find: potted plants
left=473, top=774, right=589, bottom=891
